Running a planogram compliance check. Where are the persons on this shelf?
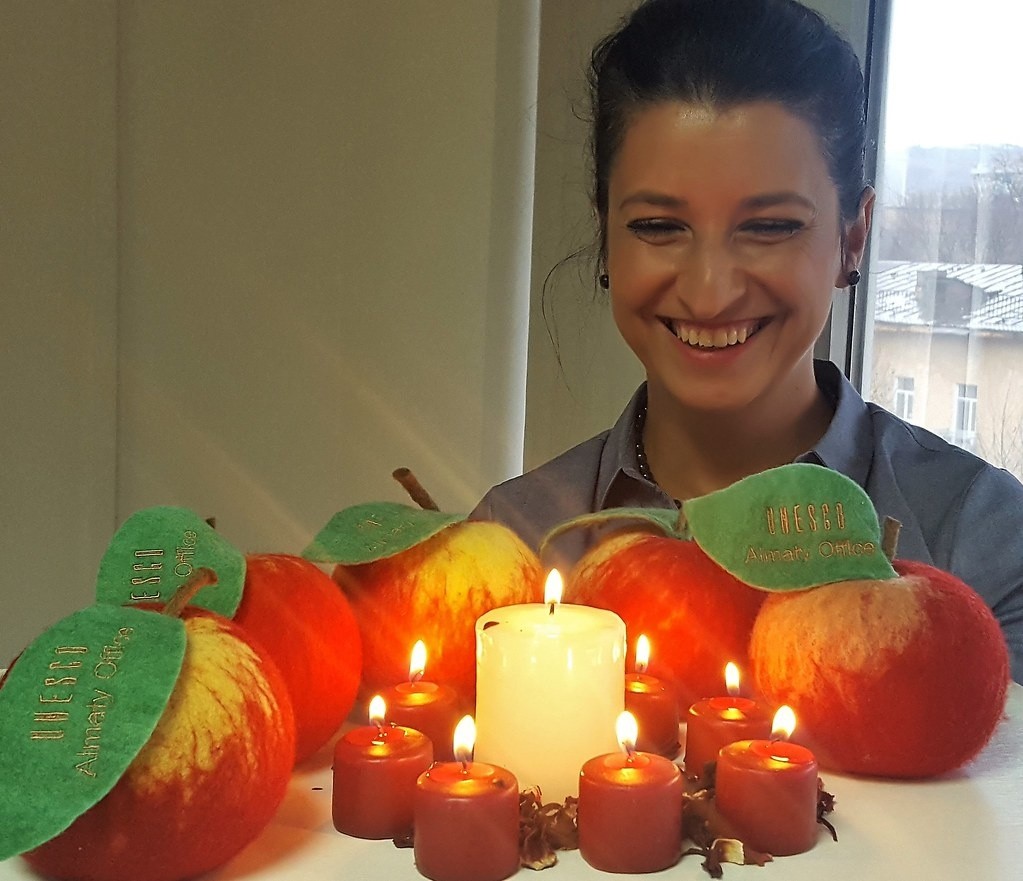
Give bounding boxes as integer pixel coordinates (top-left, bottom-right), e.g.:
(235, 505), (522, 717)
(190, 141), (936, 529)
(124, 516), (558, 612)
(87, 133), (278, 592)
(469, 0), (1023, 685)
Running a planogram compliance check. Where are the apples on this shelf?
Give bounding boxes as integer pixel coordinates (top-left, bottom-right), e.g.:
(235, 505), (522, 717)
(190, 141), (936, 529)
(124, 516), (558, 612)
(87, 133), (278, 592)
(0, 467), (1009, 881)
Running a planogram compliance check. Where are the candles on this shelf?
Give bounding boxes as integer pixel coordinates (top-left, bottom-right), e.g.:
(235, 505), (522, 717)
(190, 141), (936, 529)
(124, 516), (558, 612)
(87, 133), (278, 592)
(687, 661), (770, 782)
(621, 631), (682, 762)
(715, 703), (818, 857)
(578, 710), (685, 874)
(331, 694), (435, 840)
(473, 568), (628, 806)
(381, 638), (450, 760)
(414, 712), (521, 881)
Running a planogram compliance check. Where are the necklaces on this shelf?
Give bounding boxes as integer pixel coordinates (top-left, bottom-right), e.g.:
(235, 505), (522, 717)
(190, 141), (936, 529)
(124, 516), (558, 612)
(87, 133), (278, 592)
(632, 387), (682, 511)
